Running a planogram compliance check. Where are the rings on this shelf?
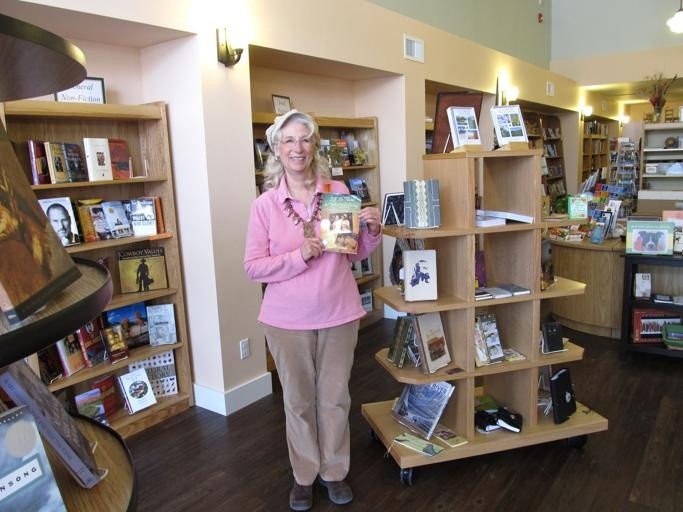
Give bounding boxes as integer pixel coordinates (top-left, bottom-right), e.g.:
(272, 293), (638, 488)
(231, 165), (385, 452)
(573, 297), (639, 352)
(375, 215), (378, 220)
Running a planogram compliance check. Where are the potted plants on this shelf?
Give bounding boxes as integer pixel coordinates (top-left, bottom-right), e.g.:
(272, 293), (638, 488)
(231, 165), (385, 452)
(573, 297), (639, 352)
(643, 73), (678, 122)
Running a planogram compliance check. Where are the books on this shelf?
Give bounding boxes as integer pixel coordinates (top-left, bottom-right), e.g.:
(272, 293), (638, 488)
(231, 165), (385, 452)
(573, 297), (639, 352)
(251, 133), (374, 314)
(116, 248), (168, 293)
(475, 209), (534, 434)
(38, 197), (165, 246)
(550, 148), (639, 244)
(626, 210), (683, 347)
(541, 323), (564, 354)
(27, 302), (177, 385)
(28, 138), (134, 187)
(447, 107), (481, 149)
(539, 238), (554, 291)
(52, 350), (178, 426)
(384, 179), (468, 457)
(524, 119), (568, 214)
(550, 368), (577, 425)
(385, 103), (683, 445)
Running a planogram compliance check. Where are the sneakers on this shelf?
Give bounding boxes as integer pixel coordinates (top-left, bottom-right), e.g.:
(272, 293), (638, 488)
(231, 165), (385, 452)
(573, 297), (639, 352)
(319, 477), (353, 504)
(290, 478), (314, 510)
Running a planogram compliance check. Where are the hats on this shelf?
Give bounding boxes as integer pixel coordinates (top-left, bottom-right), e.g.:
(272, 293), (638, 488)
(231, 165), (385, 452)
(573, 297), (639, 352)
(266, 109), (312, 151)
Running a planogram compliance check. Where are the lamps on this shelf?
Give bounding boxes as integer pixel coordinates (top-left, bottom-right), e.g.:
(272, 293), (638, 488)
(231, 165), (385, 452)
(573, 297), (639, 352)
(666, 0), (683, 34)
(216, 28), (243, 67)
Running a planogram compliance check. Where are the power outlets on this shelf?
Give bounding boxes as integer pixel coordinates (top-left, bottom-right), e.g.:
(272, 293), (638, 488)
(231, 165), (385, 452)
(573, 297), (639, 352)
(240, 338), (250, 360)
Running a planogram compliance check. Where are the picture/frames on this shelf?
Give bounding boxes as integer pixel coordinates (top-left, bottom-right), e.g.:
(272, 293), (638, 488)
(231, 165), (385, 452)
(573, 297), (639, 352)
(381, 193), (403, 227)
(54, 77), (106, 103)
(271, 94), (291, 115)
(443, 107), (482, 153)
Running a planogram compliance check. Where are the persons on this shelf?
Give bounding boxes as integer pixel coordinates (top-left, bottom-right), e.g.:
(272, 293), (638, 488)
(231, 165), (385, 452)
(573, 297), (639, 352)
(243, 108), (384, 511)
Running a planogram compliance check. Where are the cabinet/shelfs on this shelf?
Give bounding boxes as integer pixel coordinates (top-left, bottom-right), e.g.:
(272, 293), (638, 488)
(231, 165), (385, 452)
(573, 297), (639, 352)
(0, 12), (139, 512)
(252, 111), (385, 329)
(522, 112), (567, 196)
(619, 123), (683, 361)
(362, 149), (609, 470)
(582, 115), (619, 183)
(0, 99), (196, 440)
